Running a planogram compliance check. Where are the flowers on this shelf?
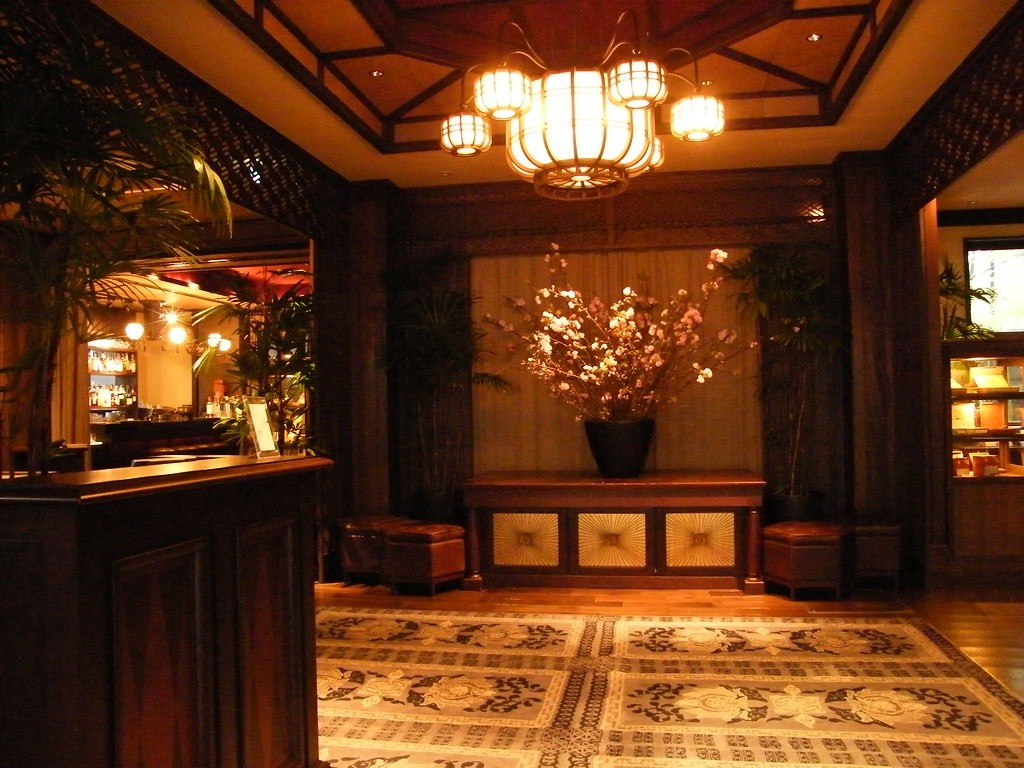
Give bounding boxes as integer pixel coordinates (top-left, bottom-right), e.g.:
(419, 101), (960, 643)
(484, 243), (807, 423)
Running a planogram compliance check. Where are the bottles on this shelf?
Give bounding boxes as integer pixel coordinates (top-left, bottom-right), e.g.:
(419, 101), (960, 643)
(88, 350), (136, 373)
(207, 395), (243, 418)
(89, 384), (136, 406)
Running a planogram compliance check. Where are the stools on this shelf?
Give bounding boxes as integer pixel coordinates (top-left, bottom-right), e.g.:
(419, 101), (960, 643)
(855, 512), (908, 607)
(337, 513), (410, 587)
(761, 521), (855, 600)
(383, 524), (467, 596)
(149, 442), (229, 455)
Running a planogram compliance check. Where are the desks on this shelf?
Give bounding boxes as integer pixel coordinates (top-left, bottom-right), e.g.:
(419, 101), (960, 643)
(0, 453), (335, 768)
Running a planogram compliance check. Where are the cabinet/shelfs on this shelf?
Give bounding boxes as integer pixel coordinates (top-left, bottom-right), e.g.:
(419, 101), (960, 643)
(944, 338), (1024, 587)
(86, 312), (147, 421)
(463, 470), (766, 595)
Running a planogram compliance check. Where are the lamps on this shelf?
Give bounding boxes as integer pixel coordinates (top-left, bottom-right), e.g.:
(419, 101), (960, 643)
(123, 300), (132, 312)
(106, 298), (113, 309)
(439, 10), (725, 202)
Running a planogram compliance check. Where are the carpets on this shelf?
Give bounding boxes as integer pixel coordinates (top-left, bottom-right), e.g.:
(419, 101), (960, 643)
(315, 607), (1024, 768)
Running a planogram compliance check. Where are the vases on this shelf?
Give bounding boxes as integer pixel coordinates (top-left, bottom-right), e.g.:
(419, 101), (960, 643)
(584, 417), (656, 478)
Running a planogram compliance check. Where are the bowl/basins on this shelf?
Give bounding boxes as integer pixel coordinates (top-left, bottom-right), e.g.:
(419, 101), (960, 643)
(134, 405), (192, 419)
(105, 412), (117, 420)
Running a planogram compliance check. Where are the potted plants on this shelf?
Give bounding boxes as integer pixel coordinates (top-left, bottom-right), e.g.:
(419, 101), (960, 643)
(713, 243), (848, 522)
(938, 250), (998, 340)
(381, 289), (522, 523)
